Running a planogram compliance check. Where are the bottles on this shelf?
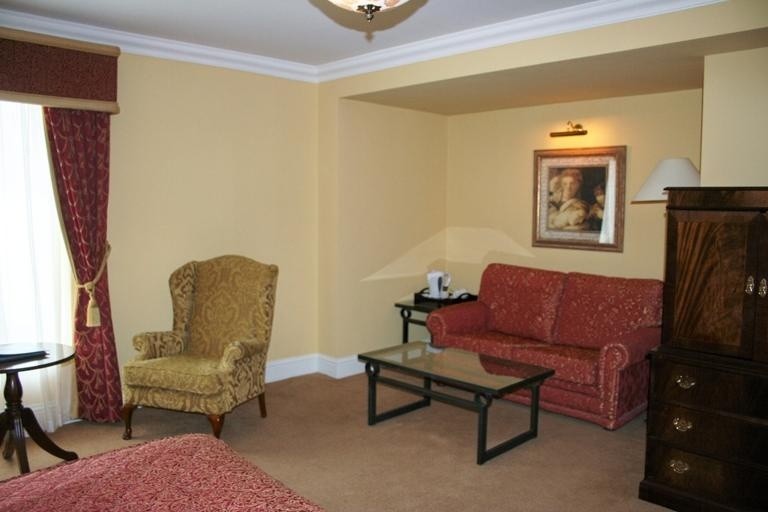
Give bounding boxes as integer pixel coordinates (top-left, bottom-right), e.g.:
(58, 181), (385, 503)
(452, 288), (468, 299)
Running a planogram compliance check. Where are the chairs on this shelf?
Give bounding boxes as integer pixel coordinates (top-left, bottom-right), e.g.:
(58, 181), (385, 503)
(120, 255), (279, 439)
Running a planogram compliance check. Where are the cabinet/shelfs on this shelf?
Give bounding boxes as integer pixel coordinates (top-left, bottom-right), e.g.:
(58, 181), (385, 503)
(661, 186), (767, 364)
(639, 348), (768, 512)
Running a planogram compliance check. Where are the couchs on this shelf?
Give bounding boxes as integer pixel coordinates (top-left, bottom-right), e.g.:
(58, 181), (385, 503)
(424, 264), (665, 431)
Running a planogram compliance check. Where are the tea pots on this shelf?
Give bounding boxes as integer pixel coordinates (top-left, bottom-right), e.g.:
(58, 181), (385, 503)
(426, 270), (452, 298)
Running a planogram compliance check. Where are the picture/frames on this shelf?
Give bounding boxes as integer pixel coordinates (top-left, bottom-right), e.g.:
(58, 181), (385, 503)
(532, 146), (626, 254)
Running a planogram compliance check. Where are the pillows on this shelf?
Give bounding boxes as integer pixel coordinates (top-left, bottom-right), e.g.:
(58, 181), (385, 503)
(552, 273), (664, 352)
(476, 265), (566, 346)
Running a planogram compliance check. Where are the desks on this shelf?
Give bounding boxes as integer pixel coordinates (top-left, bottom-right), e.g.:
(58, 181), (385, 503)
(0, 341), (77, 474)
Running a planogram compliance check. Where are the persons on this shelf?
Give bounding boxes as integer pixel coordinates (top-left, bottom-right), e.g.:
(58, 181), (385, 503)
(546, 168), (605, 232)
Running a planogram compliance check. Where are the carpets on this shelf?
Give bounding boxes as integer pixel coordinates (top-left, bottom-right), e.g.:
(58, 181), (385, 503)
(0, 431), (333, 512)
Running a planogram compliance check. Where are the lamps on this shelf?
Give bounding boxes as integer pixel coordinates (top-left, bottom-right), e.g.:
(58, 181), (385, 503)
(550, 121), (587, 136)
(327, 0), (409, 24)
(630, 157), (700, 203)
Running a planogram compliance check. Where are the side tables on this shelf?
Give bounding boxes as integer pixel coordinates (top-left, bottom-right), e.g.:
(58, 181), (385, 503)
(393, 294), (478, 343)
